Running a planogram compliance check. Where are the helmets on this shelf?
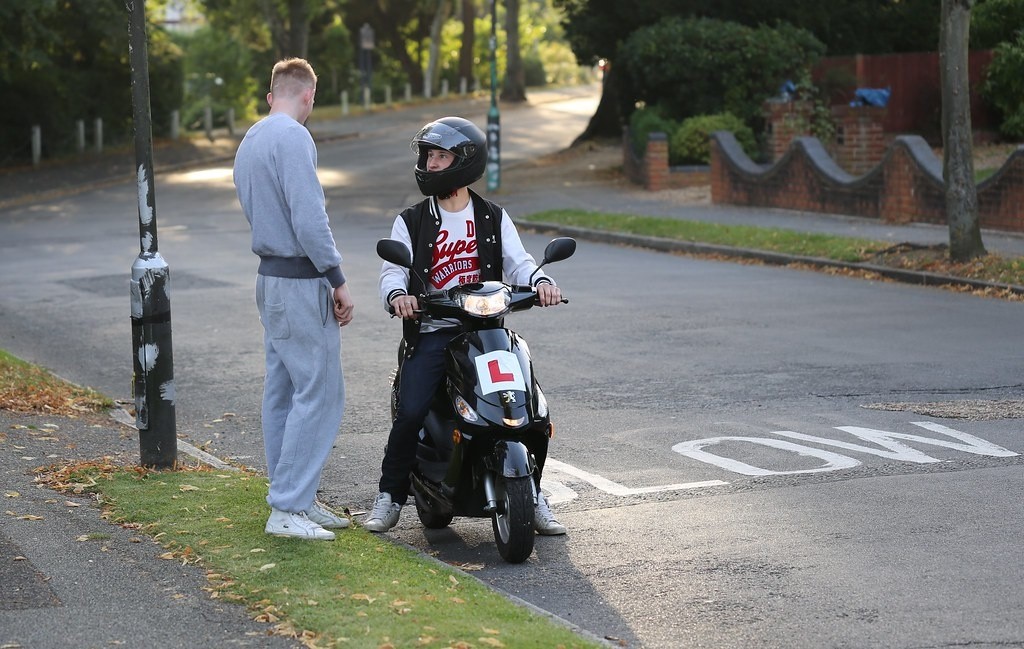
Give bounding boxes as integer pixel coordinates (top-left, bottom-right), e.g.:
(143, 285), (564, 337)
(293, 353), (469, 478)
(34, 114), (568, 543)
(410, 117), (488, 200)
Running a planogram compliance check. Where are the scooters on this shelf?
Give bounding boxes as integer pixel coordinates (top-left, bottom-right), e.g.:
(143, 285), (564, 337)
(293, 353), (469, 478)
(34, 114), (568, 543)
(375, 235), (579, 562)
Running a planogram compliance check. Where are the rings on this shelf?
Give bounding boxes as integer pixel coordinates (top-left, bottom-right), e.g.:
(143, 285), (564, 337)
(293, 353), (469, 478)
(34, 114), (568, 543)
(405, 301), (410, 305)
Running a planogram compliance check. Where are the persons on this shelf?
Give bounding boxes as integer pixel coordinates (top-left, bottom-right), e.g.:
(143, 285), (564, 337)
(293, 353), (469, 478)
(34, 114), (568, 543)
(233, 56), (352, 540)
(366, 116), (566, 536)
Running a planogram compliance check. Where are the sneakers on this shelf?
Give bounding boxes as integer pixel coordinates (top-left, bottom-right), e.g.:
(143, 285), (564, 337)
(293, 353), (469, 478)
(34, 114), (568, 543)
(307, 494), (350, 529)
(535, 492), (566, 535)
(363, 492), (402, 532)
(264, 506), (335, 540)
